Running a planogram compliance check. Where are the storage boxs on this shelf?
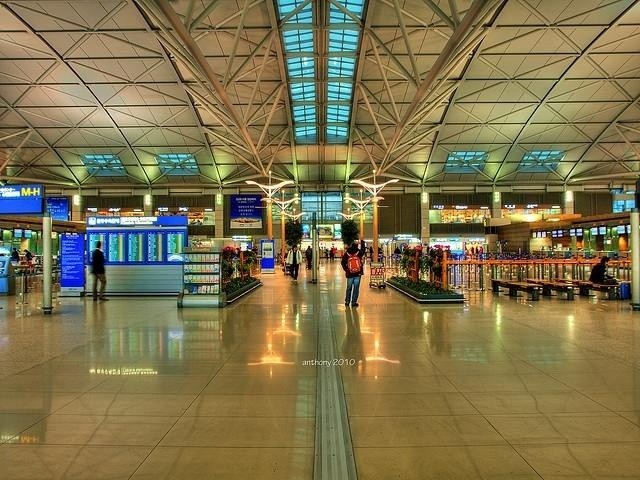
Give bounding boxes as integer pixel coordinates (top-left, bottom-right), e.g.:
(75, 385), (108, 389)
(619, 282), (632, 299)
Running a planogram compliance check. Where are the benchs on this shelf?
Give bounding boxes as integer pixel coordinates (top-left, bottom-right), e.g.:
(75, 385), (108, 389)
(490, 278), (620, 301)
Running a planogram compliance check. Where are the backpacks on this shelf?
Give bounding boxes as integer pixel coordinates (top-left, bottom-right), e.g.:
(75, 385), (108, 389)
(347, 251), (362, 273)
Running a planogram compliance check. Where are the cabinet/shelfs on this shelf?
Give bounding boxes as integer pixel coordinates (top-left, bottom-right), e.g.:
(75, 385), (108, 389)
(180, 251), (222, 296)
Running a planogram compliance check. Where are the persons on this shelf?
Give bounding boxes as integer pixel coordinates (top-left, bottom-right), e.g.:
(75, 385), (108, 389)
(12, 247), (19, 262)
(279, 244), (484, 271)
(89, 240), (110, 301)
(286, 244), (302, 283)
(588, 257), (617, 285)
(23, 247), (34, 262)
(342, 243), (365, 307)
